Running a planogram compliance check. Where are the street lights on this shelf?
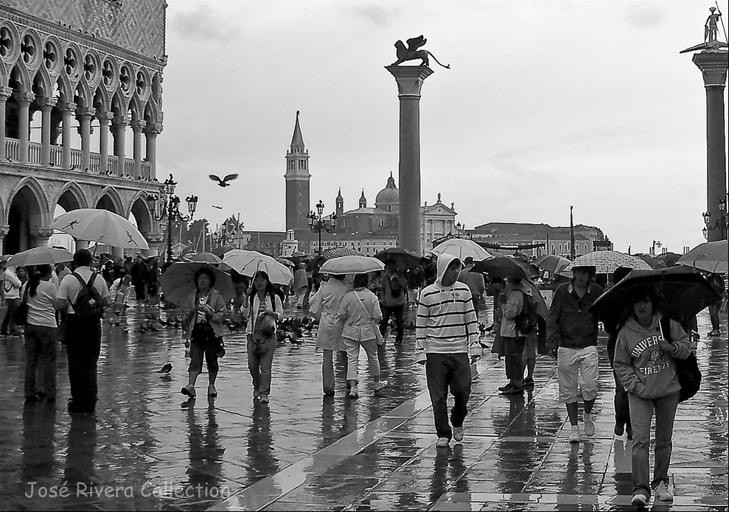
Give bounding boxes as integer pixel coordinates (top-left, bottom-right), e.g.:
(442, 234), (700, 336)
(306, 199), (339, 254)
(147, 173), (199, 300)
(447, 222), (471, 240)
(699, 198), (729, 242)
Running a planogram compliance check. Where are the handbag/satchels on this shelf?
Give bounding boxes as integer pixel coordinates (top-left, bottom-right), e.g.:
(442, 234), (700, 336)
(13, 301), (29, 327)
(373, 321), (386, 346)
(665, 350), (702, 403)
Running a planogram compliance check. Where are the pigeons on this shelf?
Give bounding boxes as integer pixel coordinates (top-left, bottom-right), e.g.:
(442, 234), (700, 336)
(478, 340), (491, 349)
(212, 206), (222, 209)
(157, 363), (172, 375)
(209, 173), (240, 187)
(139, 312), (320, 344)
(484, 323), (494, 333)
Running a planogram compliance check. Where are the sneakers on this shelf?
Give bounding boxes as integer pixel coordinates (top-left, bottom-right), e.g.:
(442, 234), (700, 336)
(569, 413), (674, 505)
(348, 388), (358, 399)
(436, 435), (450, 447)
(259, 393), (270, 404)
(181, 385), (196, 397)
(207, 384), (218, 397)
(252, 388), (261, 398)
(374, 379), (388, 391)
(497, 377), (534, 396)
(706, 327), (722, 336)
(394, 340), (402, 346)
(453, 424), (463, 442)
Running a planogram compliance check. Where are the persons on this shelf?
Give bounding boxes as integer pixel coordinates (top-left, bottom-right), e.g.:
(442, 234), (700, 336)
(704, 7), (722, 42)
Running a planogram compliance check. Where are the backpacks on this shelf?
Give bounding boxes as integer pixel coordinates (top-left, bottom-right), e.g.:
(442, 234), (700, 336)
(66, 270), (104, 323)
(508, 288), (538, 335)
